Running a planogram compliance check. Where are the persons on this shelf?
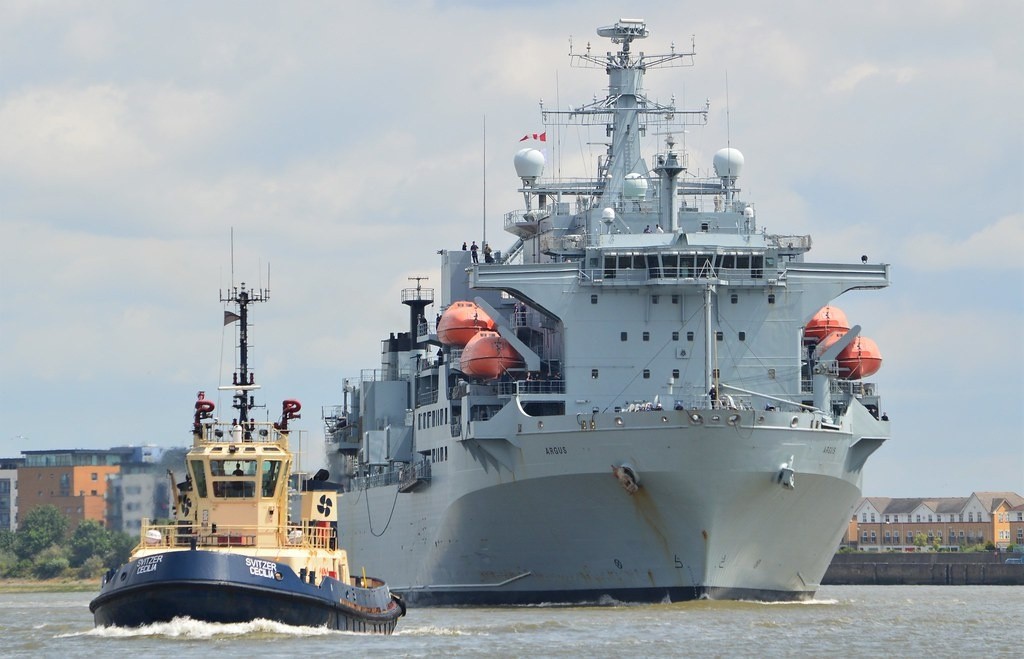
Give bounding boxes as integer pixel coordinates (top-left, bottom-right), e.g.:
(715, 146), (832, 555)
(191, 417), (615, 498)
(881, 412), (888, 421)
(437, 349), (443, 366)
(484, 244), (492, 263)
(435, 314), (441, 328)
(709, 384), (717, 409)
(470, 241), (479, 263)
(676, 404), (683, 410)
(870, 409), (879, 420)
(629, 400), (662, 411)
(461, 241), (467, 250)
(418, 314), (427, 335)
(502, 370), (562, 394)
(643, 224), (664, 233)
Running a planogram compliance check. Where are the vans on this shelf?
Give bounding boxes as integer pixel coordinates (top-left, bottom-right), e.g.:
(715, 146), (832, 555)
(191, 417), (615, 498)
(1004, 558), (1021, 564)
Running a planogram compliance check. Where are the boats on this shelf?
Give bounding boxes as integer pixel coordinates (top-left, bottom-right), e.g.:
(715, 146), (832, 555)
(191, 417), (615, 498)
(90, 273), (404, 634)
(328, 20), (892, 606)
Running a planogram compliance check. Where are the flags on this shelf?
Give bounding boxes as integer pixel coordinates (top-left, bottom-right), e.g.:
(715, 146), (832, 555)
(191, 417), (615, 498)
(520, 132), (546, 141)
